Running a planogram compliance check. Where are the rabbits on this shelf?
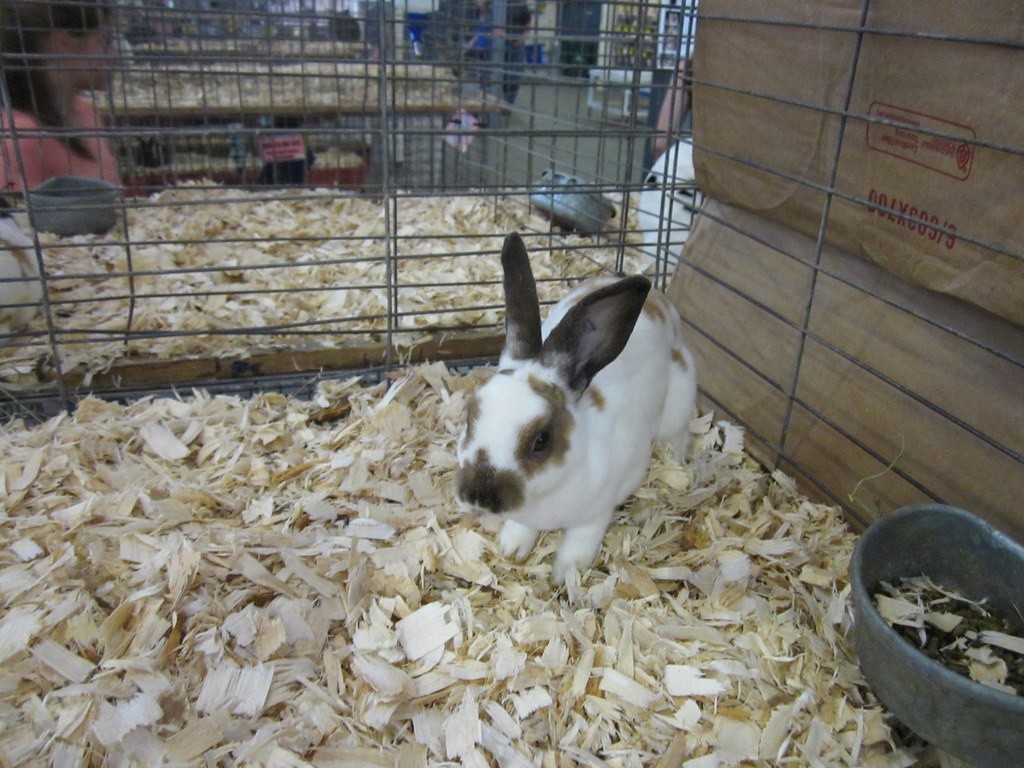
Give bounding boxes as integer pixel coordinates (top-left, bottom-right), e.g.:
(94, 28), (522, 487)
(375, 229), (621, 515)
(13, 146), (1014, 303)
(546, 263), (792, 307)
(642, 139), (706, 253)
(457, 230), (699, 587)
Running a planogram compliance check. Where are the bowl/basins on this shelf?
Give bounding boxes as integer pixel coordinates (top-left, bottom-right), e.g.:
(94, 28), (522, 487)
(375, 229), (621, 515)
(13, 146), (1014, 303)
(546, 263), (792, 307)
(531, 168), (617, 233)
(848, 503), (1024, 768)
(28, 175), (117, 235)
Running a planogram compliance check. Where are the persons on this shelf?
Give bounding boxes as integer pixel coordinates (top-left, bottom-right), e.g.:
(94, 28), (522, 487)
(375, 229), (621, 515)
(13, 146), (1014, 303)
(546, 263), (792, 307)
(0, 0), (120, 200)
(475, 0), (532, 107)
(652, 48), (693, 148)
